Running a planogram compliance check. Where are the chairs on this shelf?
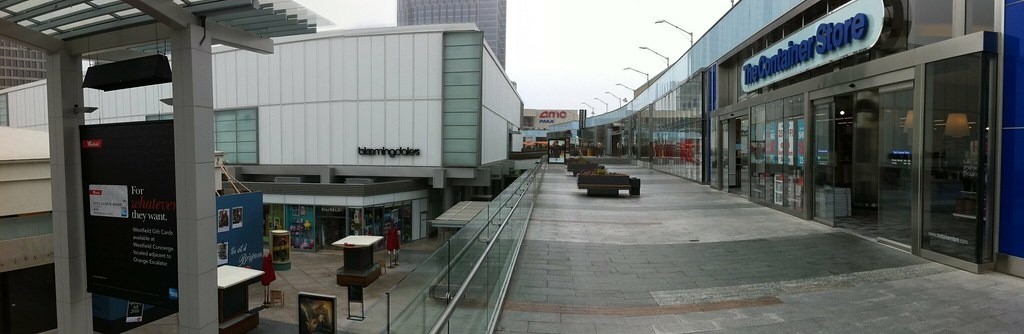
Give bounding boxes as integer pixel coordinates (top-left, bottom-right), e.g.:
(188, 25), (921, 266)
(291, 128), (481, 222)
(270, 290), (284, 308)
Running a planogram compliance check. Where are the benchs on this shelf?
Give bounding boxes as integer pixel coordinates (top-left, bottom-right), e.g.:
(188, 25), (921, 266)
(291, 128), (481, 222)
(567, 162), (631, 196)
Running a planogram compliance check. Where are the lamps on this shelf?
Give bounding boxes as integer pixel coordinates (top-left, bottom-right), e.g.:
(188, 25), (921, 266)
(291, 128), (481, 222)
(944, 113), (970, 165)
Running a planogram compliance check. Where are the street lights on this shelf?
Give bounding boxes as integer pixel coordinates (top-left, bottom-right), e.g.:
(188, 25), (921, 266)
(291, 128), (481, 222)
(605, 92), (621, 107)
(581, 102), (594, 116)
(655, 19), (693, 47)
(639, 46), (670, 68)
(616, 83), (635, 92)
(593, 97), (608, 113)
(622, 67), (649, 81)
